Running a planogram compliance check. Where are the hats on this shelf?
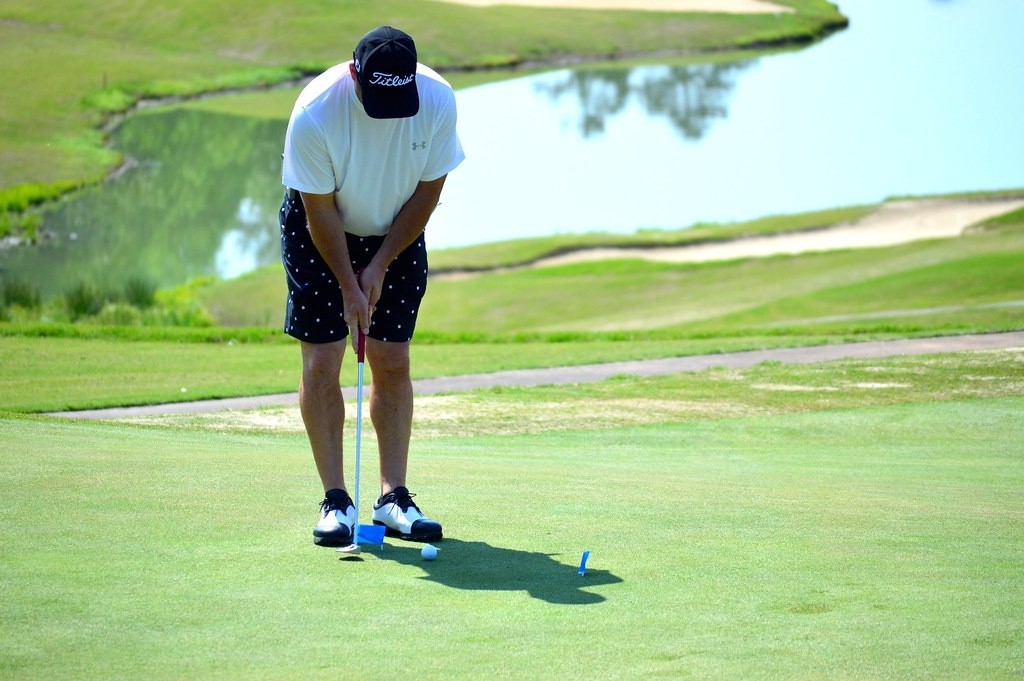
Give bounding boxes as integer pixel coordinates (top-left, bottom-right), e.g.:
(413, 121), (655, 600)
(353, 25), (420, 119)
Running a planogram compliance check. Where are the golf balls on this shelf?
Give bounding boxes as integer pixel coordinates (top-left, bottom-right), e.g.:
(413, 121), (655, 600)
(420, 543), (438, 562)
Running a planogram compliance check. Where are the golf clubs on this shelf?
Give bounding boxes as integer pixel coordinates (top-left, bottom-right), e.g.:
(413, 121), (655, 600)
(334, 323), (367, 556)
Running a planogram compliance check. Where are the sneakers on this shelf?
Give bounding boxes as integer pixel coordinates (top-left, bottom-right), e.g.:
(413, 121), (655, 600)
(312, 489), (355, 547)
(372, 486), (443, 542)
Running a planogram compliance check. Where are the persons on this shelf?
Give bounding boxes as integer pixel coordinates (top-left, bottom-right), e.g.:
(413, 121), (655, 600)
(276, 25), (468, 547)
